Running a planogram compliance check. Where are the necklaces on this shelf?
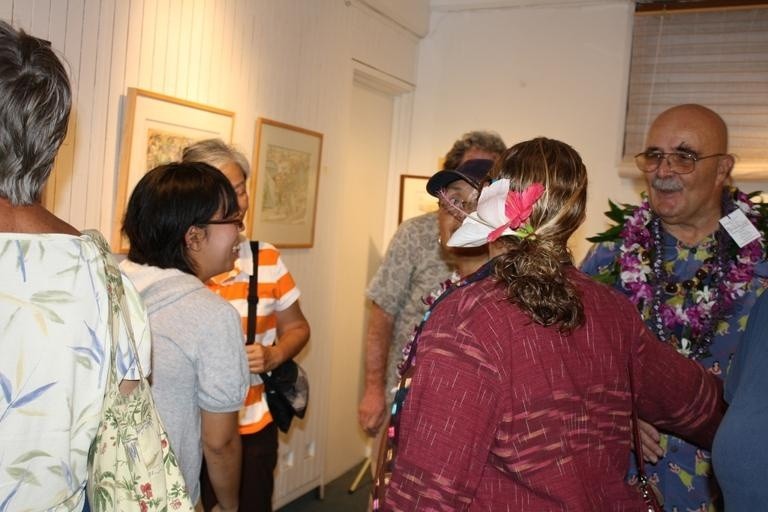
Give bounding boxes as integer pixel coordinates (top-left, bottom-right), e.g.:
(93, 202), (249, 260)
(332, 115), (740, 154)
(396, 276), (466, 369)
(619, 185), (767, 358)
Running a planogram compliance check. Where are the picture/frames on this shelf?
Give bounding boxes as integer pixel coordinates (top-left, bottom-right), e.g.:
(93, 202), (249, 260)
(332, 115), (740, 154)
(112, 86), (324, 255)
(398, 173), (440, 225)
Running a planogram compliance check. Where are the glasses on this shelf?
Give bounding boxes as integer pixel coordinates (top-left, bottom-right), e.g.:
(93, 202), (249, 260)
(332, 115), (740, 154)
(633, 150), (724, 177)
(205, 217), (245, 229)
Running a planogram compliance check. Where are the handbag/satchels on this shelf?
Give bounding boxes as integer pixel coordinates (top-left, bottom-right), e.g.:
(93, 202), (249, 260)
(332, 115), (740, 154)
(245, 240), (309, 434)
(83, 230), (195, 511)
(628, 355), (661, 512)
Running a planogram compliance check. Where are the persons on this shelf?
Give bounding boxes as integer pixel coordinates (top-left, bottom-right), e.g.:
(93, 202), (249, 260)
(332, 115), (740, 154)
(182, 135), (312, 511)
(371, 158), (499, 512)
(359, 130), (507, 476)
(0, 20), (153, 512)
(578, 103), (768, 512)
(713, 291), (768, 512)
(115, 161), (253, 511)
(384, 137), (728, 512)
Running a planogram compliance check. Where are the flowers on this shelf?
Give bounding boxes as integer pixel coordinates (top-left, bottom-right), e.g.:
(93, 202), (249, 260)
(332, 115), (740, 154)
(436, 177), (545, 250)
(585, 178), (767, 363)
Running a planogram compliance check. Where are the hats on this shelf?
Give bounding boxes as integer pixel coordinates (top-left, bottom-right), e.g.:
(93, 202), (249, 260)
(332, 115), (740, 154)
(425, 158), (492, 198)
